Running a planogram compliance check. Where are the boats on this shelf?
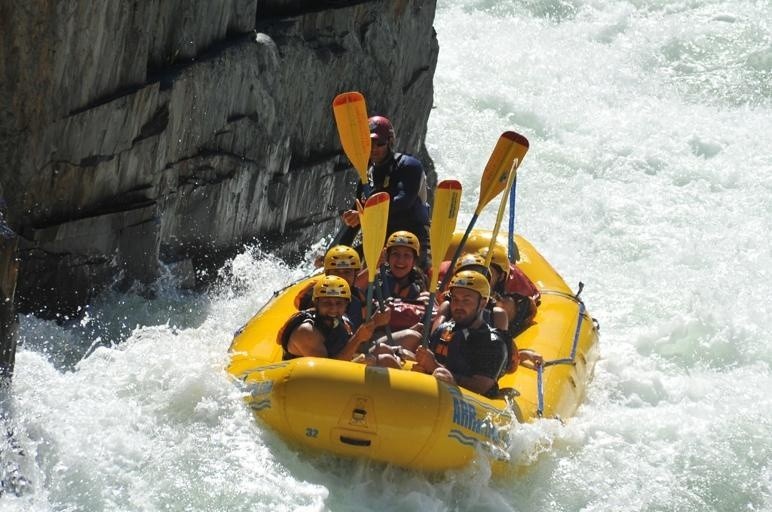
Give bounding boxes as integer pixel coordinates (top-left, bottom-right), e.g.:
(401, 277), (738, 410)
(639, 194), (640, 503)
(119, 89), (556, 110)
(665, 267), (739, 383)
(225, 231), (601, 485)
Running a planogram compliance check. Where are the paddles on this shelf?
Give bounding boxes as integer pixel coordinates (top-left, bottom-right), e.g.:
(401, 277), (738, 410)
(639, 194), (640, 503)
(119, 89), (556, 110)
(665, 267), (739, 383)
(438, 130), (529, 293)
(355, 197), (394, 346)
(422, 180), (462, 349)
(363, 191), (390, 355)
(333, 92), (391, 297)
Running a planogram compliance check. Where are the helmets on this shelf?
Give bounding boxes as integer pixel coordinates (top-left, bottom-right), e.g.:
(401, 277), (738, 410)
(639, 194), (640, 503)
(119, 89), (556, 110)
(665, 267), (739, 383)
(312, 274), (353, 306)
(386, 230), (421, 257)
(364, 114), (396, 146)
(450, 245), (510, 317)
(323, 243), (362, 274)
